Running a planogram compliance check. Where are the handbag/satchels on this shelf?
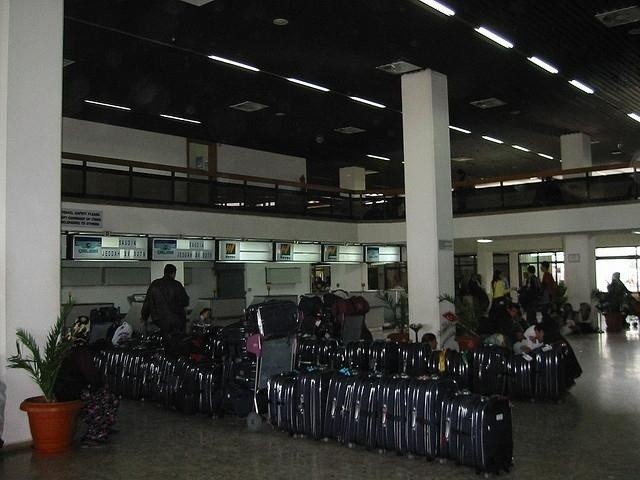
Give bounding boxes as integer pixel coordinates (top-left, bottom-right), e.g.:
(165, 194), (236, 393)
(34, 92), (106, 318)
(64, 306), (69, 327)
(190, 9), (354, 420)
(245, 289), (371, 341)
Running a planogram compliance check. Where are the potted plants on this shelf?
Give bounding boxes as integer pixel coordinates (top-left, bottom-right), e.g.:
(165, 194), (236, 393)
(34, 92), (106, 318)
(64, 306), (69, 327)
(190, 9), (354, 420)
(373, 287), (411, 342)
(434, 280), (487, 355)
(591, 281), (629, 332)
(1, 290), (98, 456)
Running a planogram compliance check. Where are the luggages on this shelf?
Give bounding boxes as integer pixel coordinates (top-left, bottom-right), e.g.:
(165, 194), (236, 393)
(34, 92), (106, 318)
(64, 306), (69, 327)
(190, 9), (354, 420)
(93, 323), (261, 418)
(267, 373), (515, 478)
(291, 336), (560, 404)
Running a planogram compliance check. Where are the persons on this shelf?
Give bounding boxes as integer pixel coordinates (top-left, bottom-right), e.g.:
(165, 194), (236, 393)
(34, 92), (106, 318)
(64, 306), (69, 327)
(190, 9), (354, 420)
(607, 272), (632, 294)
(141, 264), (190, 339)
(192, 308), (215, 336)
(421, 261), (594, 395)
(454, 169), (475, 191)
